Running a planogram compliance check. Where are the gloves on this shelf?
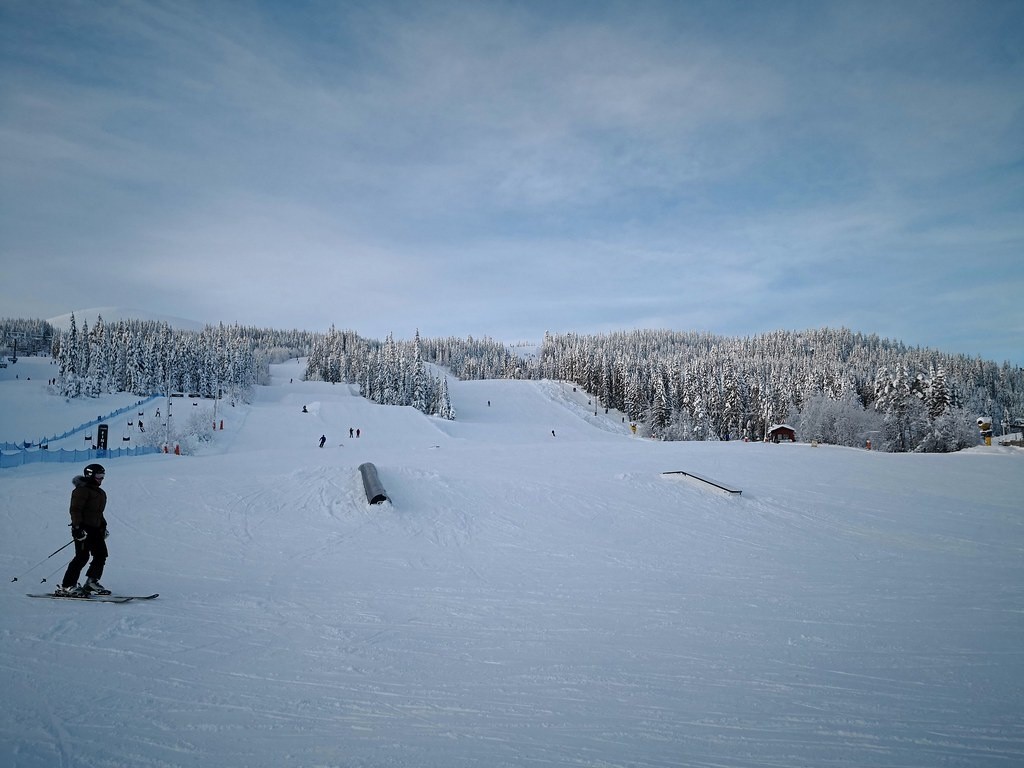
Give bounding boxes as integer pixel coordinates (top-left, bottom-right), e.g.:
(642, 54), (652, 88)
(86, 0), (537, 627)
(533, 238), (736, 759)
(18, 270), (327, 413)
(104, 529), (110, 539)
(73, 526), (89, 542)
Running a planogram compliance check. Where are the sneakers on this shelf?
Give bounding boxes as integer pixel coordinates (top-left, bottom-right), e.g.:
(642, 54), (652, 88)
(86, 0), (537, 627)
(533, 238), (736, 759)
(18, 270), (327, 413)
(61, 586), (86, 596)
(83, 577), (111, 595)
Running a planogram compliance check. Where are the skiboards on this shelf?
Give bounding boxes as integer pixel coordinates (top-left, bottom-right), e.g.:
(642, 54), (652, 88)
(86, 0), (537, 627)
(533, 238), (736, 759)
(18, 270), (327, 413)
(24, 592), (161, 604)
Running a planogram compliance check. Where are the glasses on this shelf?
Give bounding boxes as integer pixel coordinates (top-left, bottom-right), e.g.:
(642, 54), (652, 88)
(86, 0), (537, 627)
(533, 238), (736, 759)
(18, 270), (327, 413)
(95, 473), (105, 479)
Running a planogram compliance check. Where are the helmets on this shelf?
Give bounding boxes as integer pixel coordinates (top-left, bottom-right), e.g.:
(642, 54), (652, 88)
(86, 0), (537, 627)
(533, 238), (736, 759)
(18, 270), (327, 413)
(84, 464), (106, 479)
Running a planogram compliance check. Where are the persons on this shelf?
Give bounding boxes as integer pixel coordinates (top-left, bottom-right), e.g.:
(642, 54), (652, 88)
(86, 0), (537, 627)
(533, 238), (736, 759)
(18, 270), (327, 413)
(319, 435), (326, 447)
(138, 420), (145, 432)
(155, 408), (161, 417)
(231, 399), (234, 407)
(54, 464), (112, 598)
(349, 428), (354, 438)
(356, 429), (360, 438)
